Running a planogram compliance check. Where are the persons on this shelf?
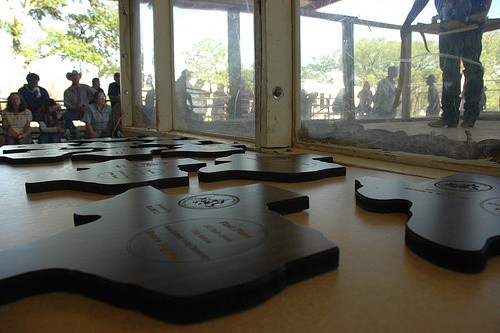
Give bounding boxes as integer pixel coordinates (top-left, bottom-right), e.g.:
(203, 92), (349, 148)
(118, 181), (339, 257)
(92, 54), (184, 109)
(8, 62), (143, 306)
(58, 70), (98, 141)
(18, 71), (50, 121)
(83, 91), (112, 139)
(178, 69), (194, 88)
(424, 74), (441, 116)
(175, 78), (194, 114)
(427, 0), (492, 129)
(374, 66), (399, 119)
(144, 74), (155, 110)
(88, 78), (107, 104)
(36, 98), (65, 144)
(192, 79), (210, 120)
(211, 83), (230, 122)
(2, 92), (33, 145)
(356, 81), (374, 118)
(107, 72), (121, 107)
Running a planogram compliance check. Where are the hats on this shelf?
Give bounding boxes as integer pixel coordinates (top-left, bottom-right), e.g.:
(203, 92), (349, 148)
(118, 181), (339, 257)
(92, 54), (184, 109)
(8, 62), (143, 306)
(424, 74), (437, 83)
(178, 69), (194, 80)
(114, 73), (120, 80)
(66, 70), (82, 81)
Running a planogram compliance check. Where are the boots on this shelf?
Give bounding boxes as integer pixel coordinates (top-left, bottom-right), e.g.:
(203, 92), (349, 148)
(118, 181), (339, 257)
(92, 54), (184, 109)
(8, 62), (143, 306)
(462, 68), (484, 127)
(426, 70), (461, 127)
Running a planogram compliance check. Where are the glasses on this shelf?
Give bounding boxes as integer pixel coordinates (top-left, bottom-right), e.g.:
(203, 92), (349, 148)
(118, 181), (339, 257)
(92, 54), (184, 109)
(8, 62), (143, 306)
(96, 96), (106, 99)
